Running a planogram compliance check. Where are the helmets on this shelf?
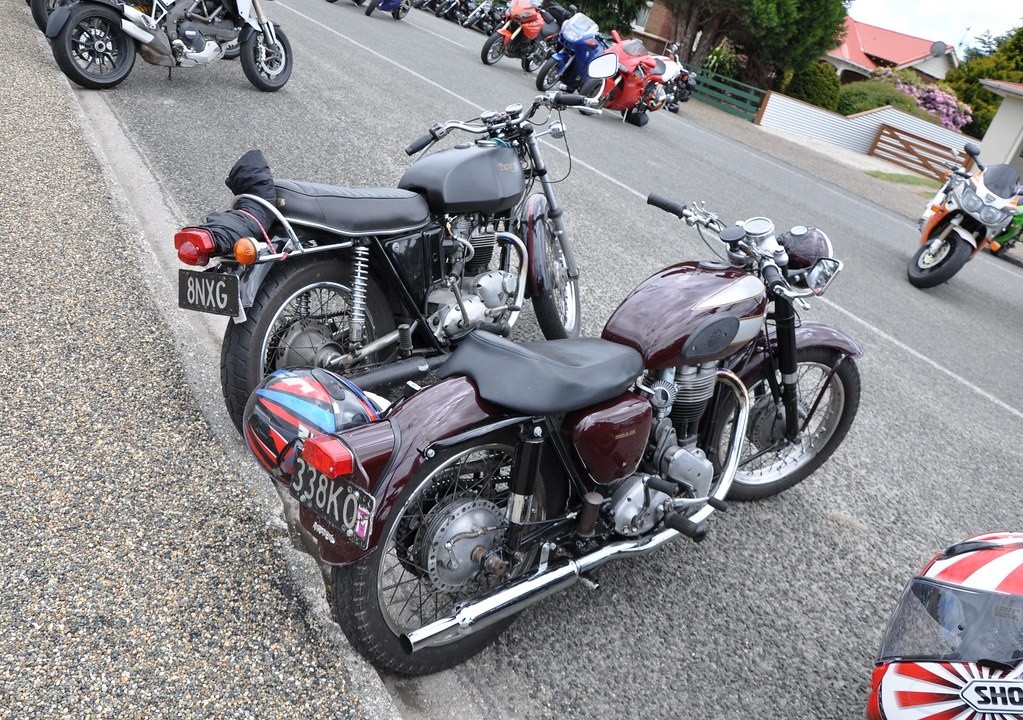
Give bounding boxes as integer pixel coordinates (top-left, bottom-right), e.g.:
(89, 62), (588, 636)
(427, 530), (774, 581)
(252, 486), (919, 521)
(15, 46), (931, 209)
(866, 531), (1023, 720)
(646, 84), (666, 112)
(242, 366), (383, 488)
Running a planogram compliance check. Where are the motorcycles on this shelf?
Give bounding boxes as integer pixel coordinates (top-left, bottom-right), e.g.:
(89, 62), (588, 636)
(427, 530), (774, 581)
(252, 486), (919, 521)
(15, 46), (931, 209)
(26, 0), (293, 93)
(244, 197), (861, 680)
(906, 142), (1023, 292)
(359, 2), (700, 128)
(168, 88), (593, 443)
